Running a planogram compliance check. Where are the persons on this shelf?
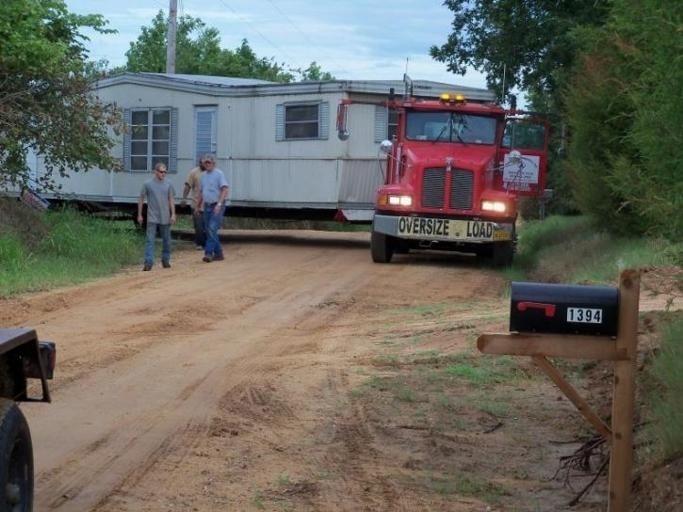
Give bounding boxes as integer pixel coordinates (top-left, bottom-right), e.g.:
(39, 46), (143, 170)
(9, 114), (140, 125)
(180, 148), (214, 250)
(137, 163), (176, 271)
(193, 156), (229, 262)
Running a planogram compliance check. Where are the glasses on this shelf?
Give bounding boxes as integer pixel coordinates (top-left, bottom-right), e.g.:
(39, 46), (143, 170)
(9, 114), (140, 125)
(157, 169), (168, 174)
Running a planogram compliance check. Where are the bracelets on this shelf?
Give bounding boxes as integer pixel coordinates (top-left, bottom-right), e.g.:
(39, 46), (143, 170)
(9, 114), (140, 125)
(217, 202), (224, 208)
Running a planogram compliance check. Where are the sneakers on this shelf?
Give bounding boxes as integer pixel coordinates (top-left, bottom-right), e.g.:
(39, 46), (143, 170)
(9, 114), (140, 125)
(195, 245), (203, 250)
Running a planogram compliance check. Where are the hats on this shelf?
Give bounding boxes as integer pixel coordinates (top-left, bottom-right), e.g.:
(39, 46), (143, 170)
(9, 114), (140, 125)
(202, 154), (216, 164)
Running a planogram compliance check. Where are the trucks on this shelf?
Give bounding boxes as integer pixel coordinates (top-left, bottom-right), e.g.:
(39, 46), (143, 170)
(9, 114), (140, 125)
(336, 96), (550, 263)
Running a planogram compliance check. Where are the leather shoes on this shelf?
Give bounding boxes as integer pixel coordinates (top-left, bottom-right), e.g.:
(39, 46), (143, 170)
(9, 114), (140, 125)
(142, 265), (152, 271)
(163, 263), (171, 268)
(202, 254), (224, 262)
